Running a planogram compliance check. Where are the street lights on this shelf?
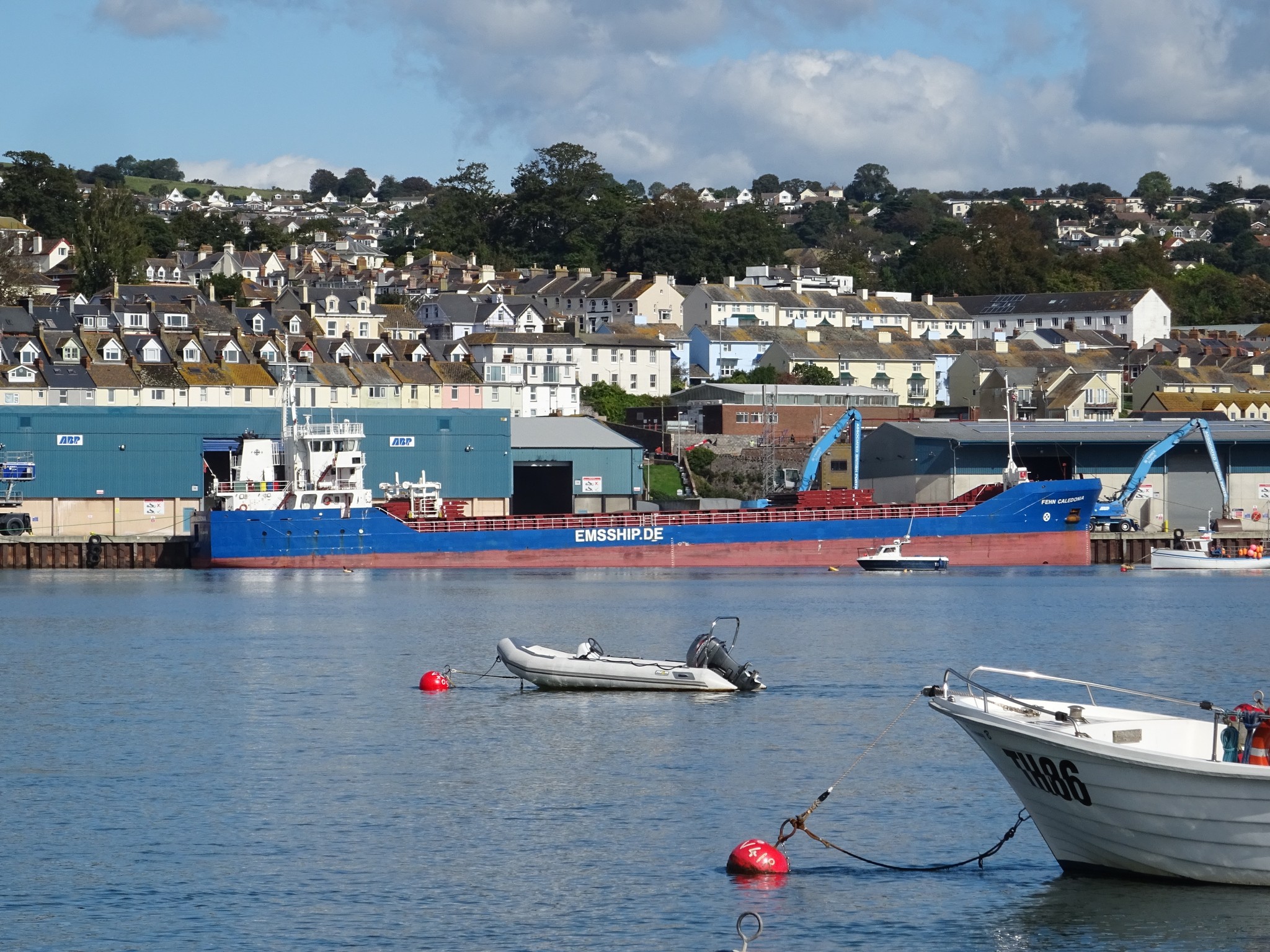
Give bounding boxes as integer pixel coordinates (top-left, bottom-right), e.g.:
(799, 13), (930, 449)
(678, 411), (683, 464)
(644, 458), (650, 501)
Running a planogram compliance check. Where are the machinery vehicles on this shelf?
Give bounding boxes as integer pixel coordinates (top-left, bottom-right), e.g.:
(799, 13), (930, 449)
(1088, 418), (1229, 532)
(740, 406), (862, 509)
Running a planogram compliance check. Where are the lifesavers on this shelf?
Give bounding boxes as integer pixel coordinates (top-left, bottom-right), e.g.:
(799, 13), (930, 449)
(1253, 513), (1260, 520)
(1229, 702), (1265, 763)
(1249, 706), (1270, 766)
(1174, 543), (1184, 550)
(89, 535), (101, 546)
(90, 554), (101, 563)
(240, 504), (247, 511)
(90, 546), (102, 554)
(1174, 529), (1184, 538)
(324, 497), (331, 505)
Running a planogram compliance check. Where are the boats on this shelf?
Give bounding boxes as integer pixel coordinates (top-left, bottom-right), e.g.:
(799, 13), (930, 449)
(855, 513), (949, 571)
(211, 335), (1102, 568)
(921, 666), (1270, 891)
(497, 616), (767, 693)
(1150, 506), (1270, 569)
